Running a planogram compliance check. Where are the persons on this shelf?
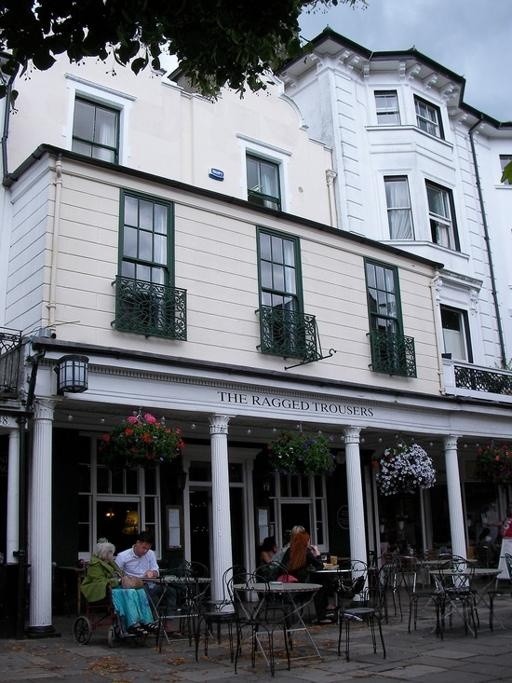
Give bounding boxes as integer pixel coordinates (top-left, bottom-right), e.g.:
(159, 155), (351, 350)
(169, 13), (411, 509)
(266, 524), (308, 582)
(257, 535), (277, 567)
(376, 521), (391, 565)
(393, 519), (412, 568)
(114, 530), (183, 638)
(493, 502), (511, 527)
(274, 530), (344, 625)
(478, 526), (500, 569)
(79, 540), (160, 636)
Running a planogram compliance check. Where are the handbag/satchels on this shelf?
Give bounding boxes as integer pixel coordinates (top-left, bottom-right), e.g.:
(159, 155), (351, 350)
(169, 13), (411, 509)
(120, 575), (144, 590)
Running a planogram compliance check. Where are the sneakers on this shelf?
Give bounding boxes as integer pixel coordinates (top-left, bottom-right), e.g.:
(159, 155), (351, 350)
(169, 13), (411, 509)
(144, 622), (160, 632)
(128, 625), (148, 637)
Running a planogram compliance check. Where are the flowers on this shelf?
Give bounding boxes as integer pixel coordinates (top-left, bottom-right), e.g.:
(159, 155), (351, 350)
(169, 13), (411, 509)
(269, 428), (336, 476)
(104, 410), (186, 466)
(468, 441), (512, 484)
(375, 440), (438, 497)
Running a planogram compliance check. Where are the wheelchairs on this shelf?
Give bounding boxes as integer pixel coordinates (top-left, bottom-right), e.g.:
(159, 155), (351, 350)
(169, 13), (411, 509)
(73, 558), (151, 648)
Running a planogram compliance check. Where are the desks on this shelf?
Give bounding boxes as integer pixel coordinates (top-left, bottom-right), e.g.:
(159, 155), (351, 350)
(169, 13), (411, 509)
(136, 575), (215, 644)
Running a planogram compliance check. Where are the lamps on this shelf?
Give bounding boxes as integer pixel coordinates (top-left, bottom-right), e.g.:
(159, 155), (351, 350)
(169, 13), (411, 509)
(54, 353), (88, 396)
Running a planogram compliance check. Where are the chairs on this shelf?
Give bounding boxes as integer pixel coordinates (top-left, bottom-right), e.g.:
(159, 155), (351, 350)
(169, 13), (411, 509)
(156, 545), (512, 677)
